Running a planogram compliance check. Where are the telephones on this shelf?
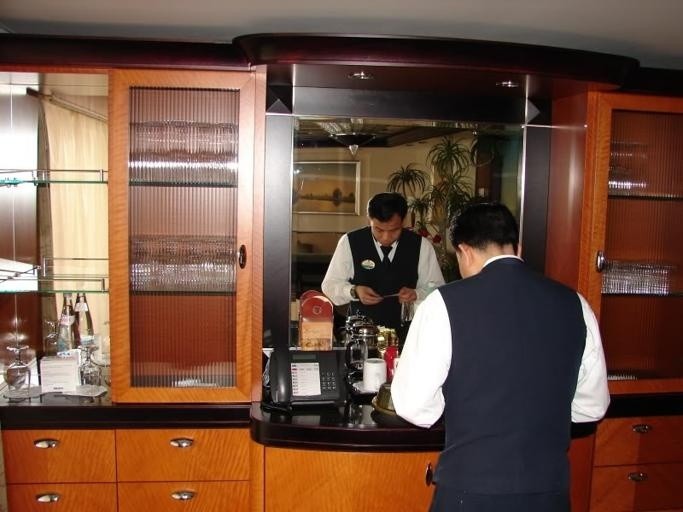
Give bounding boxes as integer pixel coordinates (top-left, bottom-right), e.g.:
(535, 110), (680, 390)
(260, 347), (347, 413)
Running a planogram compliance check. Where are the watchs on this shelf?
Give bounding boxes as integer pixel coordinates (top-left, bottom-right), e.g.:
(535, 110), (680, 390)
(350, 285), (359, 299)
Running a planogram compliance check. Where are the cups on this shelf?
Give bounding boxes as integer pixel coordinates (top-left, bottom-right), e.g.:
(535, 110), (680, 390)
(77, 345), (101, 391)
(376, 382), (402, 411)
(364, 358), (386, 390)
(5, 345), (30, 396)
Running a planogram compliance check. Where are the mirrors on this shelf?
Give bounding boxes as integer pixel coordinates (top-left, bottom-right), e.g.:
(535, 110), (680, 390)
(288, 115), (525, 359)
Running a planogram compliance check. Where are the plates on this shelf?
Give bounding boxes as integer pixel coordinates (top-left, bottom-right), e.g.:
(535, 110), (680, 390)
(371, 396), (397, 417)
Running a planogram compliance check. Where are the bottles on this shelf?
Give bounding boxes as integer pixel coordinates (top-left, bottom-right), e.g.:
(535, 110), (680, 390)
(57, 293), (80, 355)
(74, 293), (94, 348)
(384, 336), (398, 384)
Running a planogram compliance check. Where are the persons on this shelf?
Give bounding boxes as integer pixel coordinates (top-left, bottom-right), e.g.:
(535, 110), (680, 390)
(319, 192), (445, 346)
(390, 202), (611, 512)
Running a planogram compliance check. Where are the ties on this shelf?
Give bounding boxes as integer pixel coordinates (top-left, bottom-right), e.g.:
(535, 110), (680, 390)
(381, 245), (392, 265)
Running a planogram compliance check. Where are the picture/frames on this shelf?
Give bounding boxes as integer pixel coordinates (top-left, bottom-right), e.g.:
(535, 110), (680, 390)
(293, 159), (361, 217)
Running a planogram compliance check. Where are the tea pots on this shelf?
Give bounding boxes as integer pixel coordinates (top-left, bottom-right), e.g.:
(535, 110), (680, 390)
(346, 316), (381, 373)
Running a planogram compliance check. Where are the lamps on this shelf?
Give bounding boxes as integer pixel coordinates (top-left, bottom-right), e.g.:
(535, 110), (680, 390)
(313, 118), (384, 160)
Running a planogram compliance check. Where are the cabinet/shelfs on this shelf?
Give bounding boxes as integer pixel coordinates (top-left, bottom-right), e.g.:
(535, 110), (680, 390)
(0, 424), (118, 512)
(263, 445), (449, 511)
(568, 413), (682, 511)
(111, 64), (265, 407)
(0, 63), (118, 392)
(544, 91), (681, 398)
(113, 401), (255, 511)
